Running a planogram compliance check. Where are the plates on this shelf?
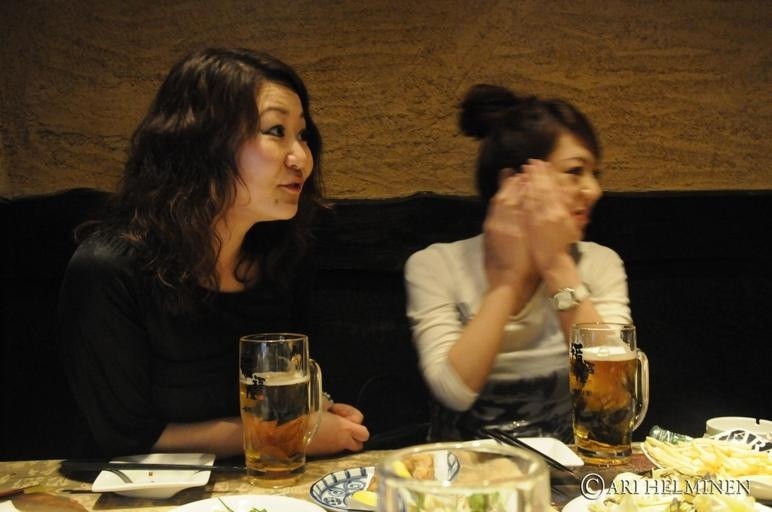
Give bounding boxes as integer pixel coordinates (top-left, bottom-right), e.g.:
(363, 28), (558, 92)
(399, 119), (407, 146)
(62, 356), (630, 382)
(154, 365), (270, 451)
(168, 493), (328, 512)
(310, 465), (406, 512)
(640, 439), (772, 501)
(560, 484), (772, 512)
(91, 452), (216, 501)
(460, 436), (585, 477)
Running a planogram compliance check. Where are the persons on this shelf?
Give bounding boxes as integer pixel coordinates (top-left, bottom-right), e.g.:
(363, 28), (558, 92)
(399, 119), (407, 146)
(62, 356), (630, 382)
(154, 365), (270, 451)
(404, 79), (631, 440)
(51, 45), (420, 458)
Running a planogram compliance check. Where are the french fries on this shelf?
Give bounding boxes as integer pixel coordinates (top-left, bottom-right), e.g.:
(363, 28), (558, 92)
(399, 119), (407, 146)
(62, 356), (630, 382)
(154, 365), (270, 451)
(643, 436), (772, 479)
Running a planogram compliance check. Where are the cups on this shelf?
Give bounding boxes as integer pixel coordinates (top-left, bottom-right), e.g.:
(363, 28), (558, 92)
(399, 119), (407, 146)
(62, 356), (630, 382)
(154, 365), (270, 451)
(238, 331), (323, 480)
(568, 320), (650, 467)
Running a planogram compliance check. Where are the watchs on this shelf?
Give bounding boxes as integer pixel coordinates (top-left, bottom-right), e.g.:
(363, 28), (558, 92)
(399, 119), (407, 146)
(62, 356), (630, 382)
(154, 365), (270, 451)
(553, 286), (587, 310)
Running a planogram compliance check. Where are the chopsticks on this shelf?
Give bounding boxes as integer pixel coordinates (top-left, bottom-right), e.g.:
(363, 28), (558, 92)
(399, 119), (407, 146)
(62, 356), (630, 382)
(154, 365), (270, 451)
(60, 461), (251, 473)
(477, 425), (575, 474)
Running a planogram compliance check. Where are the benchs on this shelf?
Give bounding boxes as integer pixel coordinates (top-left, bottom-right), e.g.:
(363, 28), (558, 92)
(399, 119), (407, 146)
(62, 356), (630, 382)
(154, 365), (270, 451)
(1, 188), (772, 459)
